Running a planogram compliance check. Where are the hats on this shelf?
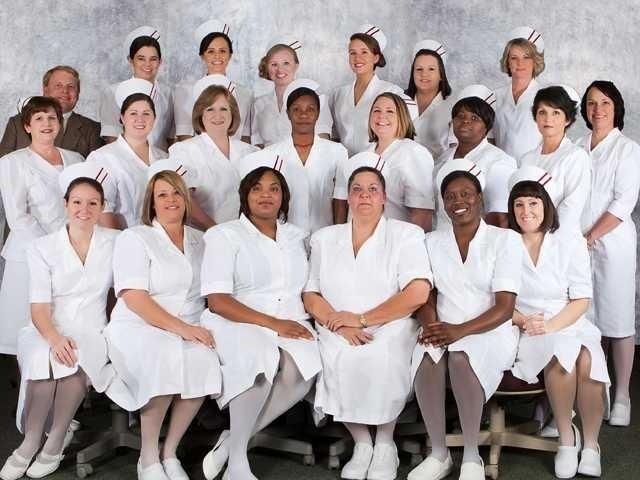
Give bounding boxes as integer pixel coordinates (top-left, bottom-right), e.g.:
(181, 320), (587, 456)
(113, 78), (160, 109)
(342, 149), (393, 195)
(263, 36), (305, 65)
(370, 85), (421, 132)
(541, 82), (582, 112)
(191, 74), (239, 106)
(283, 77), (326, 110)
(146, 160), (191, 186)
(58, 162), (117, 207)
(436, 157), (487, 198)
(452, 83), (498, 118)
(15, 95), (33, 113)
(500, 26), (544, 56)
(412, 38), (448, 67)
(234, 150), (296, 198)
(193, 20), (237, 55)
(353, 23), (388, 53)
(122, 26), (165, 65)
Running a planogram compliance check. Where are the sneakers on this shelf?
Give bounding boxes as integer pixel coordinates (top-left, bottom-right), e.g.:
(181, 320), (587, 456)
(26, 437), (66, 479)
(554, 421), (581, 479)
(458, 455), (485, 480)
(221, 466), (257, 480)
(406, 448), (453, 480)
(0, 438), (43, 480)
(541, 409), (576, 436)
(135, 456), (169, 480)
(608, 396), (632, 426)
(578, 439), (602, 477)
(367, 441), (400, 480)
(160, 451), (189, 480)
(202, 428), (230, 480)
(341, 441), (372, 480)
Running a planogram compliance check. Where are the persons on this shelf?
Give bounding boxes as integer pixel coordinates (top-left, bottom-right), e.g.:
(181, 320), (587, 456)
(519, 86), (593, 437)
(330, 23), (406, 157)
(579, 82), (639, 427)
(302, 165), (433, 480)
(407, 171), (522, 480)
(250, 79), (348, 246)
(406, 39), (454, 161)
(1, 65), (100, 241)
(426, 84), (519, 231)
(118, 25), (174, 153)
(87, 77), (169, 230)
(1, 182), (126, 480)
(173, 18), (252, 145)
(252, 36), (335, 150)
(343, 92), (434, 236)
(0, 97), (86, 430)
(492, 28), (543, 162)
(105, 169), (222, 480)
(171, 74), (261, 233)
(200, 165), (324, 480)
(505, 182), (612, 480)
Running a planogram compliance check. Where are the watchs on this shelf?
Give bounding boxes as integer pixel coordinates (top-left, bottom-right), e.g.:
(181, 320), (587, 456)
(360, 314), (367, 329)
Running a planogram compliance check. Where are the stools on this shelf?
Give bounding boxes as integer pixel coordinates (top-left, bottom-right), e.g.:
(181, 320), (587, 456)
(328, 399), (426, 470)
(428, 374), (568, 478)
(73, 390), (169, 476)
(246, 418), (319, 468)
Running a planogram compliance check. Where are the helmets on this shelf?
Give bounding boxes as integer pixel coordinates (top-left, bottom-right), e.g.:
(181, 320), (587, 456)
(507, 166), (561, 209)
